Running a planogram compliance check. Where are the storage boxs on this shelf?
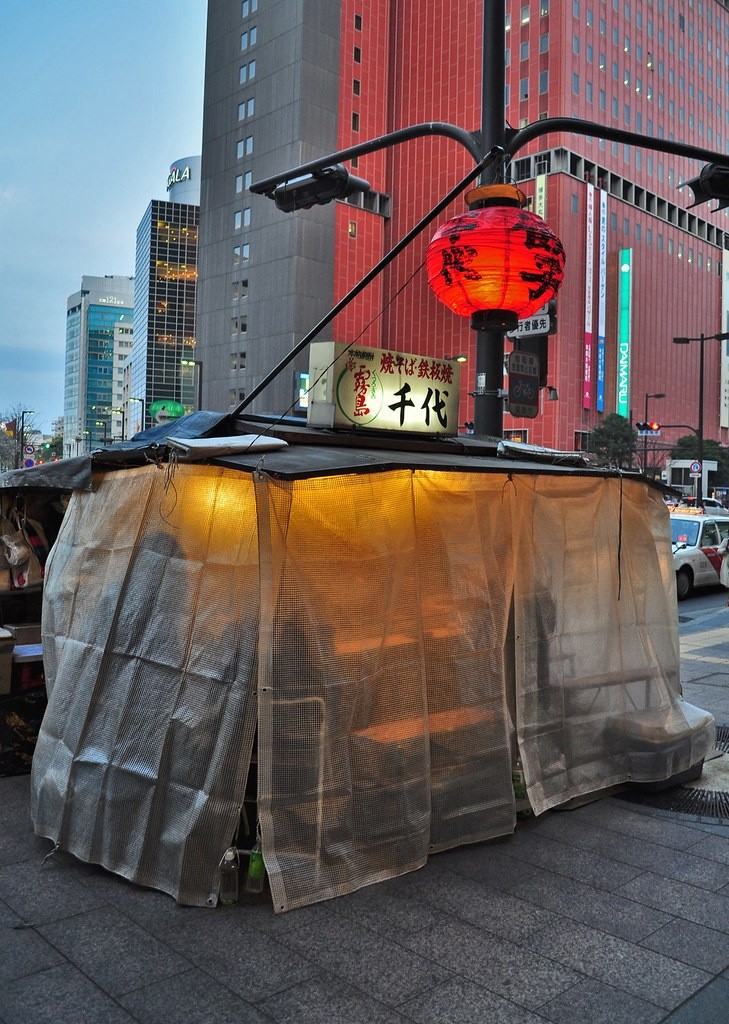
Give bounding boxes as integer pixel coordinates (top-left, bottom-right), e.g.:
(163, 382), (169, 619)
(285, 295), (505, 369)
(605, 702), (714, 793)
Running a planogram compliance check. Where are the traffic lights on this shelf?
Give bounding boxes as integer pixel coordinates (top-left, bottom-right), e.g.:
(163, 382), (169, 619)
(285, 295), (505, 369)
(636, 422), (661, 431)
(274, 163), (370, 213)
(676, 162), (729, 214)
(42, 443), (53, 448)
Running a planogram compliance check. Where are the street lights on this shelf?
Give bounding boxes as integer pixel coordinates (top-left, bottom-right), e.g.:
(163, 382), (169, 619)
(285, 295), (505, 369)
(17, 410), (34, 469)
(182, 359), (203, 411)
(128, 398), (144, 431)
(83, 430), (92, 452)
(644, 392), (666, 474)
(97, 421), (106, 446)
(112, 410), (124, 441)
(673, 332), (729, 506)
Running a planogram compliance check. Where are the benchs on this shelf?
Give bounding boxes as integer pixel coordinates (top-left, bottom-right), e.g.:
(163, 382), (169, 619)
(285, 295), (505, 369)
(348, 704), (503, 821)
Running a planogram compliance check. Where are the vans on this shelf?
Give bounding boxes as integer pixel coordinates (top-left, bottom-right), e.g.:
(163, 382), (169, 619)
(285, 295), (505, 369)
(679, 497), (729, 516)
(669, 514), (729, 600)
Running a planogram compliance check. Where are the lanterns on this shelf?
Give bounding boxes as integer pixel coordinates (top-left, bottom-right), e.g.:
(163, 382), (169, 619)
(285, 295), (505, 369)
(426, 183), (567, 331)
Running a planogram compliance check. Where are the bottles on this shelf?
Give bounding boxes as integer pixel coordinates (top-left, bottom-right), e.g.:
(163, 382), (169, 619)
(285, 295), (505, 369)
(218, 851), (239, 907)
(246, 836), (265, 894)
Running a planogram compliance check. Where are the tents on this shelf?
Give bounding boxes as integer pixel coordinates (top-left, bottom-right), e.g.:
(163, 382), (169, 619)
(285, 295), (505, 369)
(0, 410), (717, 915)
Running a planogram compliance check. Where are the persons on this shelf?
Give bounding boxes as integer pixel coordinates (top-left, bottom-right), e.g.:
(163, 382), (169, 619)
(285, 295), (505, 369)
(717, 528), (729, 607)
